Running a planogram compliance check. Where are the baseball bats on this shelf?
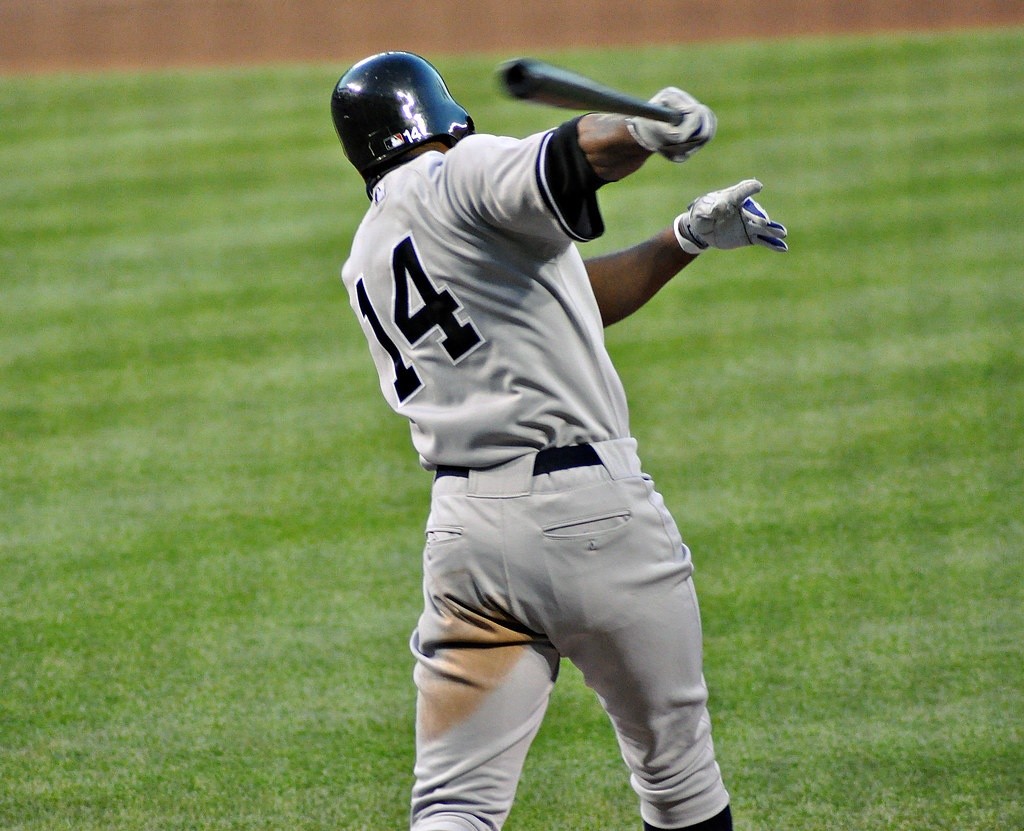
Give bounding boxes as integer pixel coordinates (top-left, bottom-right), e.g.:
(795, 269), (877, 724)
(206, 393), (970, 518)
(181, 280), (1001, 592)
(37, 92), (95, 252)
(503, 59), (685, 127)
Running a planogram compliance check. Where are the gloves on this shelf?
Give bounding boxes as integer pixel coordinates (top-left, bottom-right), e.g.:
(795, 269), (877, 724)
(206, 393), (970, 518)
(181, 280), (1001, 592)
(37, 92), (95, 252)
(625, 86), (717, 162)
(674, 179), (788, 255)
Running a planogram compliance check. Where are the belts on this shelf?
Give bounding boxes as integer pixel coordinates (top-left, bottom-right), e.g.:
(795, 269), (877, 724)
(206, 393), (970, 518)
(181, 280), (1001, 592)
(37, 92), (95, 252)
(435, 444), (602, 478)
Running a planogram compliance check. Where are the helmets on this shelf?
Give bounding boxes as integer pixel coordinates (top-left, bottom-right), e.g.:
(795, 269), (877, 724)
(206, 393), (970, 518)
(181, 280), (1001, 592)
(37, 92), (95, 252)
(331, 51), (475, 173)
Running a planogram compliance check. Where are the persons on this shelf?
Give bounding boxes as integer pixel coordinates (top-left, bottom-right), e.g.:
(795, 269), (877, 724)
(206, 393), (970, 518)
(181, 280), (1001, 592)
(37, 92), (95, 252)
(328, 52), (788, 831)
